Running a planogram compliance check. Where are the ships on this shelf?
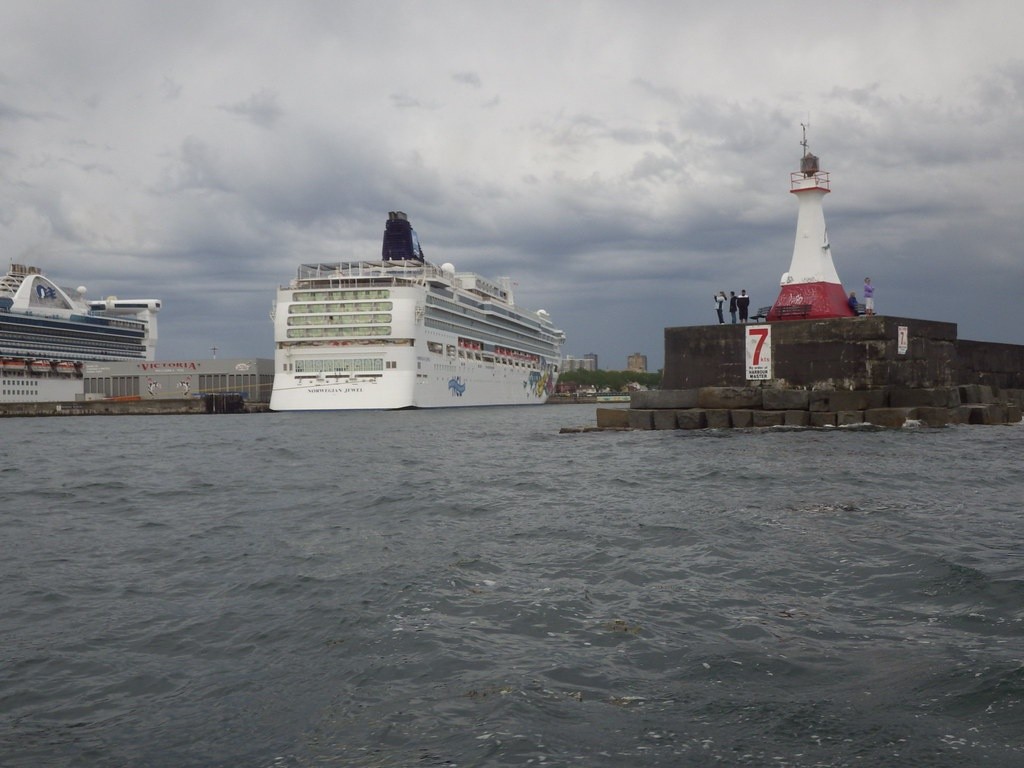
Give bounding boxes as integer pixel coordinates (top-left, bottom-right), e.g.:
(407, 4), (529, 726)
(0, 263), (162, 415)
(268, 210), (566, 412)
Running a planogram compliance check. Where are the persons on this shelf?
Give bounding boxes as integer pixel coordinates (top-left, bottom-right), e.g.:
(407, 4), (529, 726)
(848, 290), (859, 315)
(737, 290), (750, 323)
(863, 277), (876, 315)
(729, 291), (737, 323)
(714, 291), (727, 324)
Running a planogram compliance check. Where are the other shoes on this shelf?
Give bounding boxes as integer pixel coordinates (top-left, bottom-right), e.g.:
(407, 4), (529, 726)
(720, 323), (724, 325)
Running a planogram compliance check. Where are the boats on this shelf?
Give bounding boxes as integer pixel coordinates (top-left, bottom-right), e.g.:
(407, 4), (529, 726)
(57, 361), (76, 373)
(31, 359), (52, 373)
(1, 357), (26, 373)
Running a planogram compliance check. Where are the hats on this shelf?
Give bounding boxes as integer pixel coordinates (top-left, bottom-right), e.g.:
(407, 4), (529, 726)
(850, 290), (856, 296)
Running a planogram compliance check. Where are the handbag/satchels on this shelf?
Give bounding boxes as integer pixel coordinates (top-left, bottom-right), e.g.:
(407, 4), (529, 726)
(713, 301), (719, 309)
